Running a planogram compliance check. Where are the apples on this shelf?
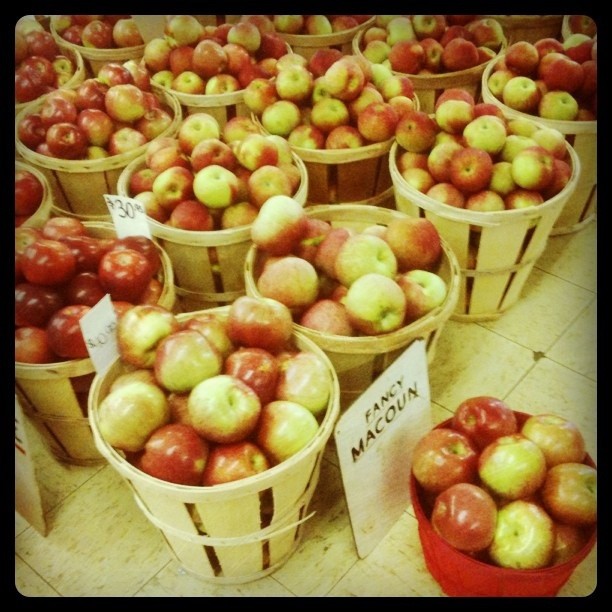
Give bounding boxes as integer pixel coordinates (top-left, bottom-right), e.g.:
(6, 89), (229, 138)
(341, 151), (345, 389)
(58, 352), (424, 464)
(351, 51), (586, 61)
(22, 60), (171, 161)
(242, 44), (415, 204)
(98, 292), (333, 570)
(362, 12), (502, 77)
(410, 394), (598, 571)
(15, 31), (73, 101)
(273, 14), (371, 40)
(395, 89), (572, 317)
(14, 216), (162, 418)
(143, 16), (289, 97)
(249, 195), (448, 339)
(128, 111), (302, 293)
(12, 167), (44, 226)
(487, 15), (596, 122)
(52, 14), (143, 49)
